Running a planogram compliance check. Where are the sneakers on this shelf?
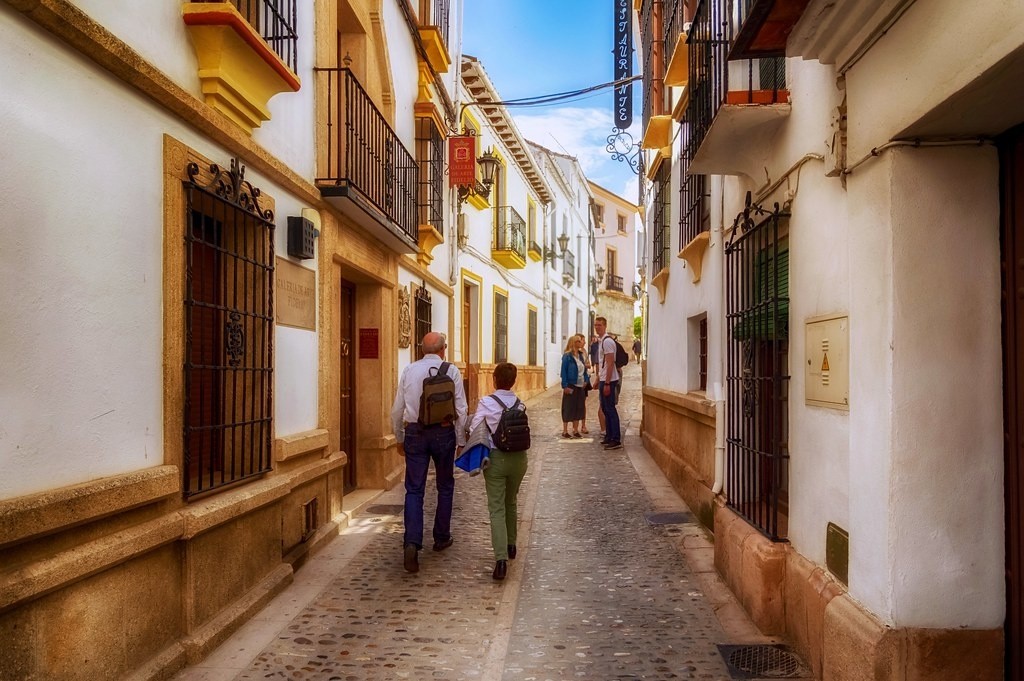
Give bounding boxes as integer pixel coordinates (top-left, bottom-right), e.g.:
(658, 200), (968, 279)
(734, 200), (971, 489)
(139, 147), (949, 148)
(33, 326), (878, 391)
(403, 543), (420, 572)
(562, 433), (583, 439)
(433, 536), (453, 551)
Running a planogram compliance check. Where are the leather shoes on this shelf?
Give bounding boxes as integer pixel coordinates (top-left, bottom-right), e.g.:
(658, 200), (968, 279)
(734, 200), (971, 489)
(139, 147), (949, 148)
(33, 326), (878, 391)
(507, 545), (516, 559)
(492, 560), (507, 580)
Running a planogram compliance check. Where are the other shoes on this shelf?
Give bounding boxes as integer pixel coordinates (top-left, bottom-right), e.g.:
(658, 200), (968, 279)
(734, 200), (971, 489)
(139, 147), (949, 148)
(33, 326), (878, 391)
(600, 440), (609, 444)
(604, 442), (621, 450)
(581, 428), (589, 434)
(597, 431), (606, 442)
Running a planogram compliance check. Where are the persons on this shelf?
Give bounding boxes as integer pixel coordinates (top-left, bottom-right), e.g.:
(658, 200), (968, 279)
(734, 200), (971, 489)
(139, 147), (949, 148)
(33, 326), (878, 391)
(392, 332), (468, 573)
(470, 362), (528, 580)
(560, 317), (642, 450)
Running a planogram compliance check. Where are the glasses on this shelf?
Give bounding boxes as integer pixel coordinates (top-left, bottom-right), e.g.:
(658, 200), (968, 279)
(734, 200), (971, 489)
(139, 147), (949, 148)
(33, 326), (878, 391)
(441, 344), (447, 350)
(575, 341), (581, 343)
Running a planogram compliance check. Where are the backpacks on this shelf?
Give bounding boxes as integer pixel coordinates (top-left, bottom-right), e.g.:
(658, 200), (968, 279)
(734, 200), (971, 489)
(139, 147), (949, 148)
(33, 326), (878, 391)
(602, 336), (628, 369)
(484, 395), (531, 453)
(418, 363), (459, 426)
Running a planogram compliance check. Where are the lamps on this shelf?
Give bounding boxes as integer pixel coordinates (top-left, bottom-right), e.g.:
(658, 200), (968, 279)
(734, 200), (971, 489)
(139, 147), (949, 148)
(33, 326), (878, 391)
(544, 234), (570, 265)
(591, 267), (606, 285)
(475, 147), (502, 198)
